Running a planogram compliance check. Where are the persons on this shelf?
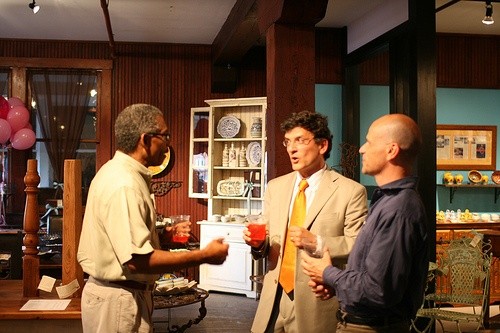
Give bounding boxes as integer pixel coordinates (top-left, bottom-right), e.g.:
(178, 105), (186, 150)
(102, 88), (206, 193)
(76, 104), (229, 333)
(300, 114), (429, 333)
(243, 110), (369, 333)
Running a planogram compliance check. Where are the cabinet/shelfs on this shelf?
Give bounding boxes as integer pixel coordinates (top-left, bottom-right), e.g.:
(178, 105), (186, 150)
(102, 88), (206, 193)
(435, 222), (500, 305)
(188, 96), (269, 298)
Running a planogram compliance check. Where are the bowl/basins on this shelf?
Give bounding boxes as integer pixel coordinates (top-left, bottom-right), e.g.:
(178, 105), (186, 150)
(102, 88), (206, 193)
(490, 170), (500, 185)
(467, 170), (482, 183)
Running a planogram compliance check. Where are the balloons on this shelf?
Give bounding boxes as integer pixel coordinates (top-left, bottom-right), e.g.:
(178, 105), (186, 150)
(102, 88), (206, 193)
(0, 95), (36, 150)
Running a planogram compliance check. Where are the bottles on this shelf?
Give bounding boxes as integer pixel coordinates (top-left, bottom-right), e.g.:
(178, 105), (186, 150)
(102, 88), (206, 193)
(193, 151), (208, 168)
(197, 171), (204, 193)
(222, 142), (229, 167)
(239, 142), (246, 166)
(229, 142), (236, 166)
(251, 117), (262, 138)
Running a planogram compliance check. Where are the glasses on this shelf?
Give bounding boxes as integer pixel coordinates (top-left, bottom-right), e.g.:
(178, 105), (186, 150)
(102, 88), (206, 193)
(281, 136), (315, 147)
(144, 132), (174, 140)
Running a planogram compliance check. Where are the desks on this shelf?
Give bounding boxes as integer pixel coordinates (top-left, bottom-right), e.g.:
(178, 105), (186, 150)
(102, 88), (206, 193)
(152, 287), (209, 333)
(0, 229), (88, 333)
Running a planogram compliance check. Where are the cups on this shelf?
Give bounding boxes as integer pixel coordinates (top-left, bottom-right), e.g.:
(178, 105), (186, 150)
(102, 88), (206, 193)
(168, 214), (191, 243)
(248, 216), (269, 241)
(302, 234), (325, 259)
(211, 213), (247, 223)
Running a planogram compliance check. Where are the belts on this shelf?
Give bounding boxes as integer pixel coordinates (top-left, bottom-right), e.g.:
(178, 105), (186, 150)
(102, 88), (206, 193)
(334, 307), (408, 326)
(108, 280), (157, 293)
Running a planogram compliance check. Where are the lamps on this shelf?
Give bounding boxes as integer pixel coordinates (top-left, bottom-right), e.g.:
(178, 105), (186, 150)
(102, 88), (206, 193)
(482, 2), (494, 25)
(29, 0), (39, 13)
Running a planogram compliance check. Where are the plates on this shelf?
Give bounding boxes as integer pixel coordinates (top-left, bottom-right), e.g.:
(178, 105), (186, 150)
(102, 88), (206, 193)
(217, 116), (241, 138)
(217, 180), (245, 196)
(246, 142), (262, 167)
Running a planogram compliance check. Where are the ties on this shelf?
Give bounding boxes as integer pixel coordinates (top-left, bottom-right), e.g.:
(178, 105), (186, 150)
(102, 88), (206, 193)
(279, 179), (309, 293)
